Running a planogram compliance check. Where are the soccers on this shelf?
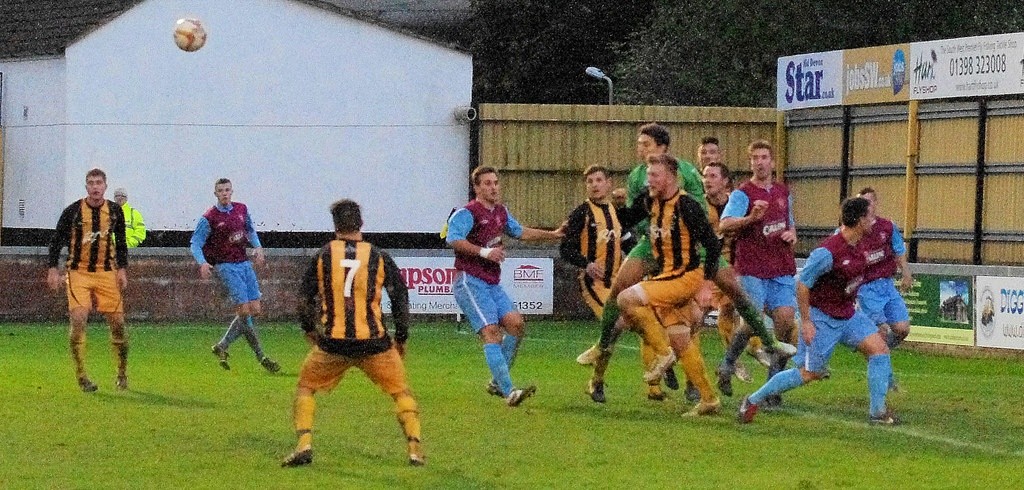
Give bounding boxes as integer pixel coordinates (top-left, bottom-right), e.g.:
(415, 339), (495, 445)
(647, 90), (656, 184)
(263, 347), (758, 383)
(173, 19), (206, 52)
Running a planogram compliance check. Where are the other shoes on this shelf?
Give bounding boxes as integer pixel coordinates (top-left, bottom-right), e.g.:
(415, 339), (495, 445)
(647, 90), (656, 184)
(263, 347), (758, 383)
(282, 446), (312, 469)
(78, 377), (99, 393)
(117, 373), (127, 389)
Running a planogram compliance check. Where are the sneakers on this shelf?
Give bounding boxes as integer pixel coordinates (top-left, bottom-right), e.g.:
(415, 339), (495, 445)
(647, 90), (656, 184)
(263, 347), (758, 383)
(715, 366), (731, 396)
(649, 390), (669, 402)
(681, 398), (723, 419)
(748, 347), (771, 367)
(644, 346), (675, 382)
(256, 355), (282, 372)
(684, 381), (698, 399)
(211, 342), (231, 371)
(585, 378), (608, 402)
(732, 362), (755, 384)
(506, 386), (536, 405)
(869, 413), (911, 428)
(737, 394), (757, 423)
(762, 401), (795, 417)
(577, 342), (614, 364)
(487, 381), (505, 399)
(662, 366), (678, 389)
(765, 341), (796, 357)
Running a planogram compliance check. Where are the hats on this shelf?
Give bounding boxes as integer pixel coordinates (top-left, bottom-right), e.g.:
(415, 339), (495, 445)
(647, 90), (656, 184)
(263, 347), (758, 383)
(113, 188), (127, 202)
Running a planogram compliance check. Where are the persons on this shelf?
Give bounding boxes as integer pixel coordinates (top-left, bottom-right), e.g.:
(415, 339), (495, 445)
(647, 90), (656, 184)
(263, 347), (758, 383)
(733, 197), (908, 426)
(447, 165), (566, 404)
(281, 199), (428, 466)
(190, 178), (282, 374)
(46, 169), (130, 391)
(558, 120), (802, 422)
(113, 186), (146, 248)
(833, 187), (912, 348)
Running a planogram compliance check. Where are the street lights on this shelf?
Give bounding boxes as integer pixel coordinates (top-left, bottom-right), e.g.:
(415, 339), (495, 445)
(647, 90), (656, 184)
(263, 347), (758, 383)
(585, 67), (614, 107)
